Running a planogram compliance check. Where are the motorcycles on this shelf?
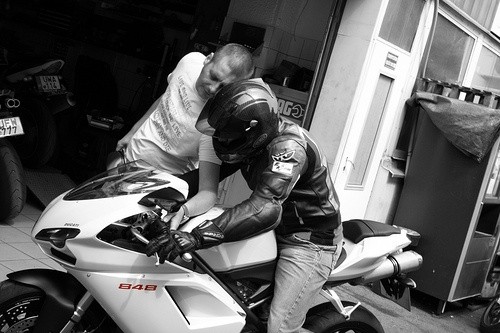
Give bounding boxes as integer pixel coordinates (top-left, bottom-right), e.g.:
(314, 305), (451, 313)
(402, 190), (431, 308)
(0, 156), (424, 332)
(480, 296), (500, 332)
(0, 59), (68, 222)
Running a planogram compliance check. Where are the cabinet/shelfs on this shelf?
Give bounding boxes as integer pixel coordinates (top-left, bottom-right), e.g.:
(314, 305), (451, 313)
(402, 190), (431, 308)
(395, 90), (500, 313)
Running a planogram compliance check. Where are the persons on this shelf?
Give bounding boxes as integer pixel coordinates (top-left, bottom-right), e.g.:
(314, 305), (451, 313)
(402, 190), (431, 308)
(5, 46), (71, 99)
(105, 42), (255, 231)
(145, 77), (344, 333)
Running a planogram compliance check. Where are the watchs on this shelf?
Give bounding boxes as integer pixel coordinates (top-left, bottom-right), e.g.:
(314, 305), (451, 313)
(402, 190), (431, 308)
(180, 204), (189, 225)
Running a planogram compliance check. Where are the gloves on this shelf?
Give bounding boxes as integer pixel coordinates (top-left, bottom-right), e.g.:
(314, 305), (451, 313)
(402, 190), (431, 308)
(146, 220), (224, 264)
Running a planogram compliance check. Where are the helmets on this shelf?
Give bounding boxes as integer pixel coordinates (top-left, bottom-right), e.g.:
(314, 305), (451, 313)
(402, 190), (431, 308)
(196, 78), (278, 165)
